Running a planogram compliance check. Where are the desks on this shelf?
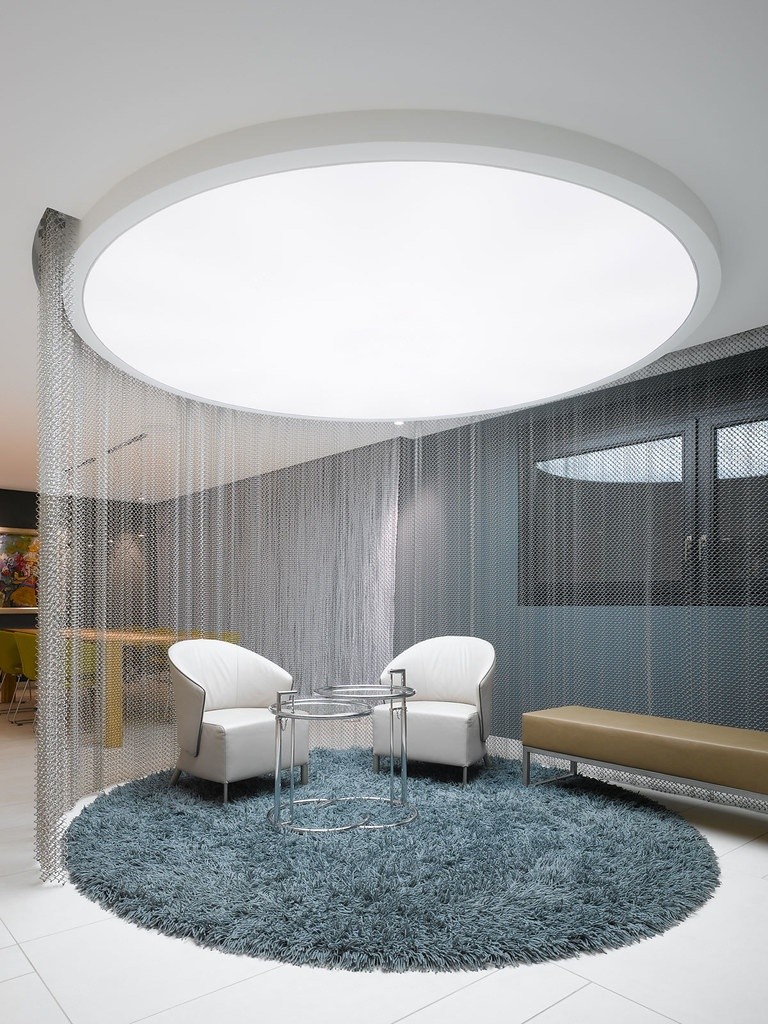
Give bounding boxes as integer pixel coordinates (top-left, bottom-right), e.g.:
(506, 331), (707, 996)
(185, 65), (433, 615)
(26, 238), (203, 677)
(270, 690), (373, 832)
(6, 629), (231, 747)
(320, 668), (416, 828)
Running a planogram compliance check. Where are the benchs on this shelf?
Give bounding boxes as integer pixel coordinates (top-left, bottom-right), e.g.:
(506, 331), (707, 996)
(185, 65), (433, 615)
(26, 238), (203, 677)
(522, 705), (768, 816)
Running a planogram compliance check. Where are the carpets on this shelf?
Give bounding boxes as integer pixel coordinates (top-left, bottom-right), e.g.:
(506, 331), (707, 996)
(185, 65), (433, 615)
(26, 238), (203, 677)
(60, 744), (722, 973)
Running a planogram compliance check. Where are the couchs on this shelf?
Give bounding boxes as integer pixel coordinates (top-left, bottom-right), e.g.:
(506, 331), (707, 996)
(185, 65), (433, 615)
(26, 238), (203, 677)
(168, 638), (307, 805)
(371, 634), (495, 782)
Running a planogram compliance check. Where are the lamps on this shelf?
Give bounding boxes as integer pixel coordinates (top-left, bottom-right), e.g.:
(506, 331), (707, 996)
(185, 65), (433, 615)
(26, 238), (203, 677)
(63, 112), (720, 423)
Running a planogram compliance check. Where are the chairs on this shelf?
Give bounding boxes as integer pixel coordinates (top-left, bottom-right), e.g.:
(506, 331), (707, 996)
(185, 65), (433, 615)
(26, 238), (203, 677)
(0, 630), (100, 738)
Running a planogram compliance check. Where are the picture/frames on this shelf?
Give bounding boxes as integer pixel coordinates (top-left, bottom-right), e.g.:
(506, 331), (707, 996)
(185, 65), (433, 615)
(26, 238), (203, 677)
(0, 527), (39, 614)
